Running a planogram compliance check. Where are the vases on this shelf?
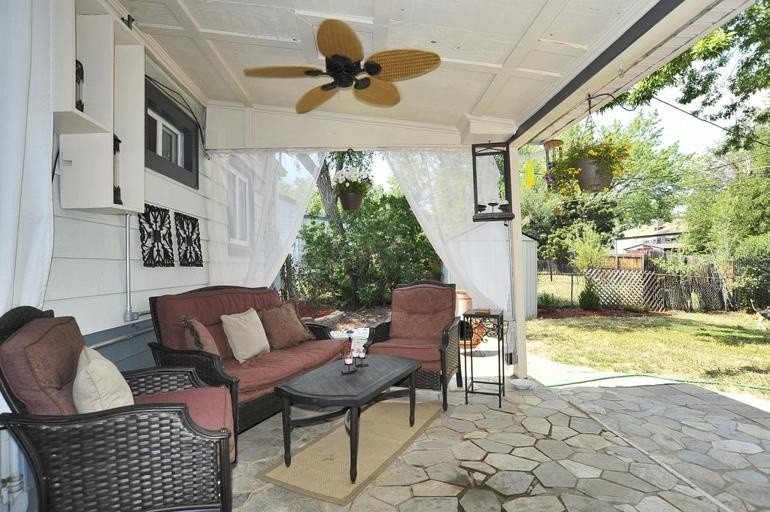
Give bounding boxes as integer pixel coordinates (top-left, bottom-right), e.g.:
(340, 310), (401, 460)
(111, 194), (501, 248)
(574, 159), (612, 193)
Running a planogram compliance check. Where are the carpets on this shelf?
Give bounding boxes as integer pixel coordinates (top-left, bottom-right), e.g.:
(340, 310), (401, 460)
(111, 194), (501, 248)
(255, 402), (443, 506)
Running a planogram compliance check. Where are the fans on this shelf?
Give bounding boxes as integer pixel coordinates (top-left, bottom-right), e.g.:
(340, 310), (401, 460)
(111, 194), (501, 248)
(243, 18), (441, 113)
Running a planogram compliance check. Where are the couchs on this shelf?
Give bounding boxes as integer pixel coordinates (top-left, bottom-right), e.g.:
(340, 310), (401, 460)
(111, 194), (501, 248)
(146, 286), (353, 434)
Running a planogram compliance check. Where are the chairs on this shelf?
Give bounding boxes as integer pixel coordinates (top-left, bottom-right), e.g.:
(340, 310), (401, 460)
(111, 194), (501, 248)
(0, 306), (235, 512)
(362, 279), (461, 411)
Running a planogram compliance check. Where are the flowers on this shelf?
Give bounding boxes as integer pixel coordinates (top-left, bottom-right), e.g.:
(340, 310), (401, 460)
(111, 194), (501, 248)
(541, 142), (640, 193)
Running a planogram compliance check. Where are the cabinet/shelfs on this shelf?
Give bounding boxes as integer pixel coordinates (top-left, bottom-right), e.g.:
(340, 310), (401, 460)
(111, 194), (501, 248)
(50, 1), (146, 216)
(463, 308), (507, 408)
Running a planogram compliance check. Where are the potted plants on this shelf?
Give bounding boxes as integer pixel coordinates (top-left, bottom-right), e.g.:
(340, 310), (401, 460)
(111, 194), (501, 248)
(332, 177), (374, 212)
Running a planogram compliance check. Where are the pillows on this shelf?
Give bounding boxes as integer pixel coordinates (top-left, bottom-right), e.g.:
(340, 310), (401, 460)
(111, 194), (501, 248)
(183, 315), (219, 355)
(221, 307), (270, 364)
(73, 345), (134, 414)
(258, 298), (313, 350)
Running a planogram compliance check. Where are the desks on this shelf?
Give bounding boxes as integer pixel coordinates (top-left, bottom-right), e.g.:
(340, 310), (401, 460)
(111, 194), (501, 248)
(274, 353), (422, 483)
